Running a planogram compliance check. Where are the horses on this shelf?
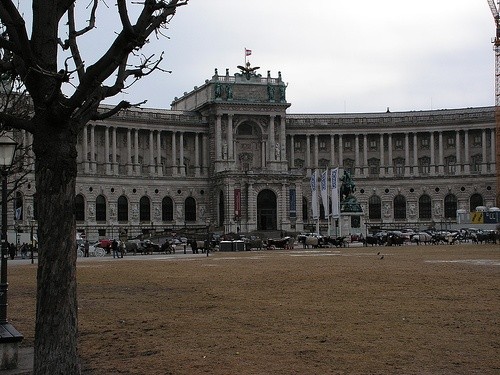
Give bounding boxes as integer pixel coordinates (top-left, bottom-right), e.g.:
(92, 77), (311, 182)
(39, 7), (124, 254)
(298, 230), (484, 249)
(187, 238), (208, 254)
(95, 240), (111, 255)
(125, 241), (138, 255)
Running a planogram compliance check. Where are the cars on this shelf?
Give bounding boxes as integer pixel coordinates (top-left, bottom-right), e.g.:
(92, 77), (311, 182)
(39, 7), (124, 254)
(351, 232), (364, 243)
(296, 233), (325, 243)
(93, 238), (163, 252)
(366, 228), (496, 246)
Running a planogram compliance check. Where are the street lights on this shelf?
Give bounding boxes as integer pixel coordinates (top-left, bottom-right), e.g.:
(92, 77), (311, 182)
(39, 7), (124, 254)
(29, 218), (35, 264)
(0, 135), (21, 325)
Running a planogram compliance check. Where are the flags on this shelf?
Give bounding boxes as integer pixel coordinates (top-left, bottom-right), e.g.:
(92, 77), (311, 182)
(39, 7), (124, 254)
(245, 50), (251, 56)
(321, 170), (329, 219)
(331, 167), (340, 218)
(311, 171), (320, 220)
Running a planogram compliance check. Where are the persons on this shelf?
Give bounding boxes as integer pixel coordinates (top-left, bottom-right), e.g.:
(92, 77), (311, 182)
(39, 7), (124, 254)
(112, 239), (120, 258)
(164, 239), (172, 254)
(119, 239), (125, 257)
(132, 239), (154, 255)
(107, 239), (111, 254)
(84, 240), (89, 257)
(8, 242), (30, 260)
(339, 170), (347, 192)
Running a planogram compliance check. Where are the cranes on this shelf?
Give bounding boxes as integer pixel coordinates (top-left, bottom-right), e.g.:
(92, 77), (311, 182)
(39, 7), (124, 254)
(486, 0), (500, 130)
